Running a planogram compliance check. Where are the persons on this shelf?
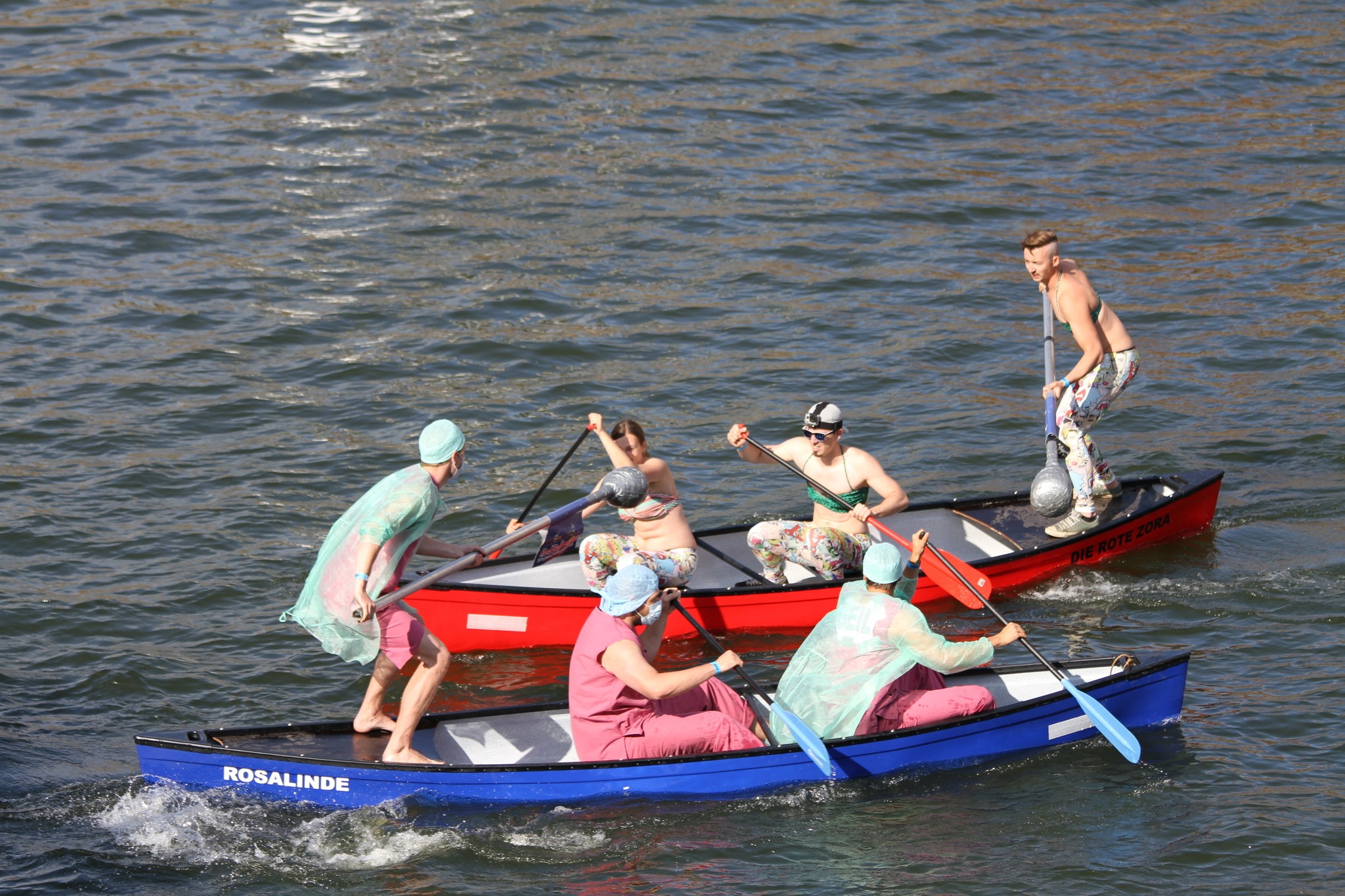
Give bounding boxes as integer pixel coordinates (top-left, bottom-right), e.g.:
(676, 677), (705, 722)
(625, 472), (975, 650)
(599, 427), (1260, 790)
(279, 419), (488, 765)
(1020, 230), (1140, 539)
(727, 401), (910, 588)
(763, 529), (1026, 747)
(506, 413), (698, 596)
(568, 563), (766, 762)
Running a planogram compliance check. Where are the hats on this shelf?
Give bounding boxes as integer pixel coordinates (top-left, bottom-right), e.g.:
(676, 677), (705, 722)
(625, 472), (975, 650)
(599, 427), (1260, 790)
(808, 402), (849, 434)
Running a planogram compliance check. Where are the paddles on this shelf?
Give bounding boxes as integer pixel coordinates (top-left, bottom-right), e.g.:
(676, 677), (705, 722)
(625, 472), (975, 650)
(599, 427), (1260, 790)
(489, 420), (605, 559)
(733, 425), (992, 609)
(918, 531), (1142, 765)
(668, 585), (831, 779)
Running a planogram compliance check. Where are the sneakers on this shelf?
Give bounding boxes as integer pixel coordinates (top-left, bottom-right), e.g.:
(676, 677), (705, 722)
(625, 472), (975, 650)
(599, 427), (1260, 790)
(1045, 508), (1100, 538)
(1073, 481), (1122, 499)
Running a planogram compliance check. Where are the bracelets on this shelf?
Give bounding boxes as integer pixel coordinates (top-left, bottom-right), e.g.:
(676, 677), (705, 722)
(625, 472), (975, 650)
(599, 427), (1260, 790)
(906, 557), (921, 570)
(1060, 378), (1070, 389)
(354, 573), (370, 582)
(710, 662), (721, 677)
(737, 441), (749, 450)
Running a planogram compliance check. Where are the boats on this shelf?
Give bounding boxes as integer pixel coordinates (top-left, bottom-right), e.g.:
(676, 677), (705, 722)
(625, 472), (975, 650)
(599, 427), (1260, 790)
(396, 464), (1226, 656)
(133, 646), (1192, 806)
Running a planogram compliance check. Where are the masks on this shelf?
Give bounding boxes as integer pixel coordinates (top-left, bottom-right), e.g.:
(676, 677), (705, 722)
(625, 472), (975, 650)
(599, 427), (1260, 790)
(635, 599), (662, 625)
(449, 452), (465, 479)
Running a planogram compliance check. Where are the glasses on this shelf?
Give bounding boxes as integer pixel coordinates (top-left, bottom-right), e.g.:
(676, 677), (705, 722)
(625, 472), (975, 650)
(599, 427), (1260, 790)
(802, 426), (834, 442)
